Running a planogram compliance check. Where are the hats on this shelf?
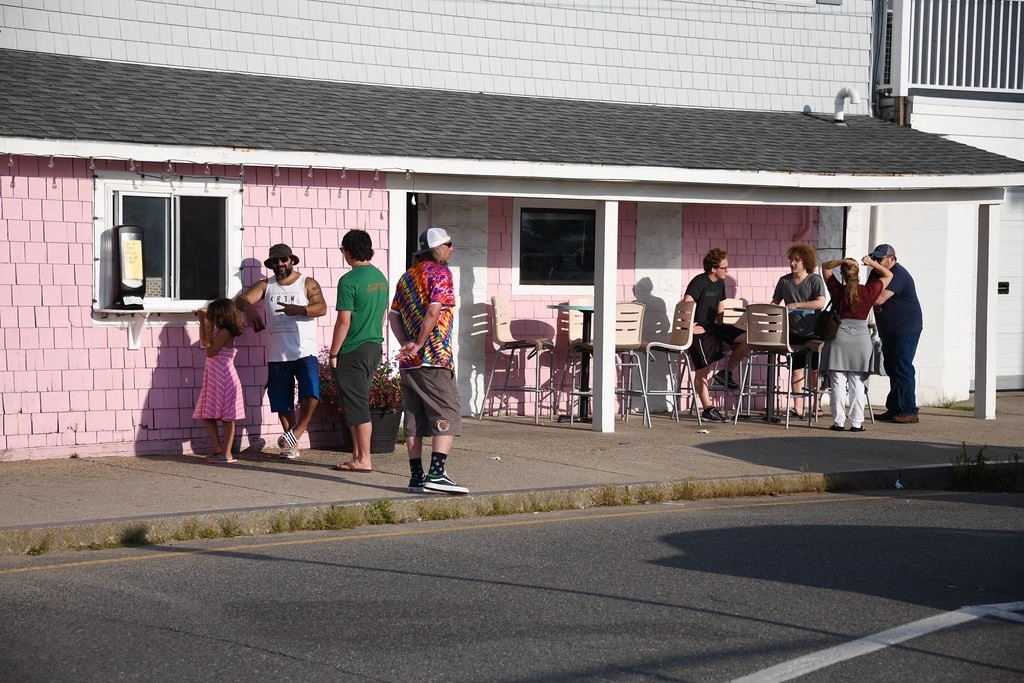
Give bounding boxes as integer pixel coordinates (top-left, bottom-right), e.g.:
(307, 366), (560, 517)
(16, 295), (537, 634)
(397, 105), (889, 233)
(263, 244), (299, 270)
(867, 243), (895, 258)
(413, 227), (451, 254)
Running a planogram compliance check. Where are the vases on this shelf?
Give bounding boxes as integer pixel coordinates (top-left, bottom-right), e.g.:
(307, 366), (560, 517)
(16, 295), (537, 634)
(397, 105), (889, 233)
(343, 408), (402, 453)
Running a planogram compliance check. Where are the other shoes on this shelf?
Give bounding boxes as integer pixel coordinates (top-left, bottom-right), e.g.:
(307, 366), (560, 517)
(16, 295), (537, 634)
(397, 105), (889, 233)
(829, 423), (844, 431)
(873, 410), (892, 420)
(892, 412), (919, 423)
(850, 424), (866, 432)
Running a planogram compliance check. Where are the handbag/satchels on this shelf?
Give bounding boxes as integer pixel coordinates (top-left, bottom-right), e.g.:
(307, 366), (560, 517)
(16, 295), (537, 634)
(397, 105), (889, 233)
(813, 308), (841, 340)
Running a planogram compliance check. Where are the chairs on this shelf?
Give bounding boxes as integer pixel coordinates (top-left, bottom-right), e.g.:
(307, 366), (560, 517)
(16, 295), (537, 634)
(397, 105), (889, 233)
(477, 295), (556, 424)
(708, 298), (782, 419)
(616, 302), (652, 430)
(624, 301), (703, 426)
(555, 297), (593, 425)
(802, 340), (875, 423)
(734, 302), (813, 431)
(668, 349), (730, 421)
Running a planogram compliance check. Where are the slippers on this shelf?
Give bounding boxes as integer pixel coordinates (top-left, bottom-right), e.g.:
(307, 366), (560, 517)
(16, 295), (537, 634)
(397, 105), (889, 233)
(212, 454), (238, 464)
(278, 423), (303, 450)
(781, 409), (804, 419)
(812, 407), (824, 418)
(332, 461), (372, 473)
(278, 446), (300, 459)
(204, 452), (213, 458)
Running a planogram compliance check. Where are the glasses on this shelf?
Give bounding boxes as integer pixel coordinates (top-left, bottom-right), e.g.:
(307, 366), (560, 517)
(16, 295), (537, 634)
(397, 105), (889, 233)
(787, 257), (803, 263)
(871, 257), (883, 263)
(269, 256), (289, 265)
(442, 241), (452, 248)
(339, 247), (344, 253)
(717, 265), (729, 272)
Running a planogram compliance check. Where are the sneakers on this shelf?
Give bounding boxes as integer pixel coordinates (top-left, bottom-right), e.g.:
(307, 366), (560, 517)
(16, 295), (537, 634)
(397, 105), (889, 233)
(407, 473), (429, 493)
(715, 370), (738, 389)
(424, 470), (469, 494)
(701, 407), (731, 423)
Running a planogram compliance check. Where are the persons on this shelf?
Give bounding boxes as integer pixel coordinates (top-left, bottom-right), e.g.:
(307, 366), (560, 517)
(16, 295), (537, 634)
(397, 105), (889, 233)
(330, 230), (389, 471)
(682, 248), (748, 422)
(865, 244), (922, 423)
(192, 298), (246, 464)
(769, 242), (826, 419)
(238, 244), (327, 458)
(822, 256), (893, 431)
(389, 227), (471, 494)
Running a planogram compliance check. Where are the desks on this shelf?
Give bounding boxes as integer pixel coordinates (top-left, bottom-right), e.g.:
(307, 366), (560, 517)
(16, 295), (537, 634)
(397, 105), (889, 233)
(733, 306), (815, 425)
(546, 305), (593, 424)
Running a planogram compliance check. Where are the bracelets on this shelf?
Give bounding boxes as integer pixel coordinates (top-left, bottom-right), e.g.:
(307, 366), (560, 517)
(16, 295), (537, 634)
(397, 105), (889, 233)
(330, 354), (337, 359)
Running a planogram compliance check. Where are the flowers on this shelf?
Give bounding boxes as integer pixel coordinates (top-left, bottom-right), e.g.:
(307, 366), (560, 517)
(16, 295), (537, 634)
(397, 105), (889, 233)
(318, 343), (404, 414)
